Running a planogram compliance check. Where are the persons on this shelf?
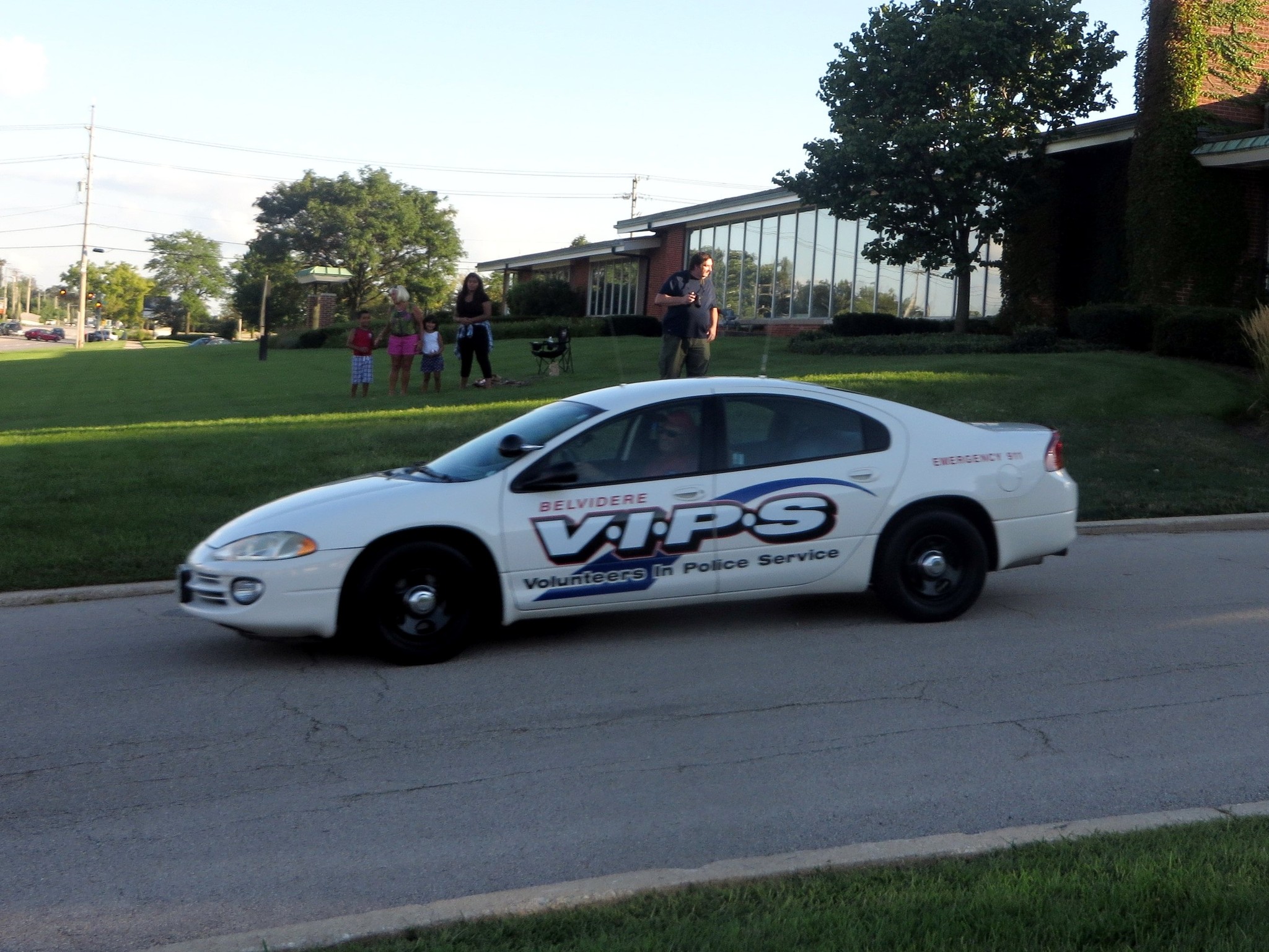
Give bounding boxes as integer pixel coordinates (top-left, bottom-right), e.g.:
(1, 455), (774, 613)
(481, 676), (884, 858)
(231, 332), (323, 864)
(374, 284), (423, 402)
(654, 252), (718, 379)
(346, 311), (379, 400)
(572, 408), (698, 485)
(417, 315), (445, 394)
(451, 273), (493, 389)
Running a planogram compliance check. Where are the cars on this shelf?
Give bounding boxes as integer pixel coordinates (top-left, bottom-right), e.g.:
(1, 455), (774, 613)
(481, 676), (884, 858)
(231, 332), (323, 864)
(24, 328), (66, 342)
(85, 329), (119, 343)
(45, 319), (57, 325)
(190, 336), (242, 346)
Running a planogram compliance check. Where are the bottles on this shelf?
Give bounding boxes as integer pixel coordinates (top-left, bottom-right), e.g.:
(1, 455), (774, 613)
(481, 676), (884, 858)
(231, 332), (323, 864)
(548, 337), (553, 342)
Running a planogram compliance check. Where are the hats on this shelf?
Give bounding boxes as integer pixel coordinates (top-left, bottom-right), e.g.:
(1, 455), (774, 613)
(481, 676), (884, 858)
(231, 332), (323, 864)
(664, 409), (693, 429)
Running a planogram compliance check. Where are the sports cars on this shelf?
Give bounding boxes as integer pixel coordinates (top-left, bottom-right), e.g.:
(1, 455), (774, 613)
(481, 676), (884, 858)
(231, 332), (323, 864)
(176, 374), (1078, 665)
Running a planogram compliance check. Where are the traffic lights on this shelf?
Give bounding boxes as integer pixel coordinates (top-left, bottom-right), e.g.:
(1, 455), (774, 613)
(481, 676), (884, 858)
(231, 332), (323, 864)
(96, 302), (101, 307)
(88, 292), (102, 298)
(46, 287), (66, 295)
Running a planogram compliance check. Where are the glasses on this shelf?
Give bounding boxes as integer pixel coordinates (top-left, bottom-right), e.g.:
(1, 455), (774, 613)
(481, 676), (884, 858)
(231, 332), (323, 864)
(656, 425), (688, 438)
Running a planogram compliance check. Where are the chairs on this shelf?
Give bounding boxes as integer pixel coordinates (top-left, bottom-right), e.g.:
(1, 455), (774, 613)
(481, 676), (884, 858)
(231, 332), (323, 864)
(528, 325), (574, 374)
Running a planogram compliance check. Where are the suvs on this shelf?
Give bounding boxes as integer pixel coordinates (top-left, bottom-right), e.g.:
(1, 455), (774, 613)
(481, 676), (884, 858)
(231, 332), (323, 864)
(0, 322), (22, 331)
